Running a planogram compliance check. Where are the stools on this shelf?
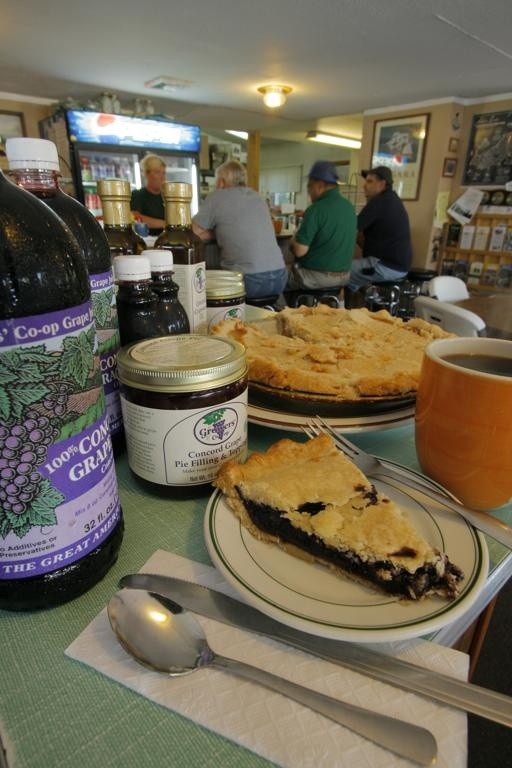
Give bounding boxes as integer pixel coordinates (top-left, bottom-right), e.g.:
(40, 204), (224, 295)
(294, 287), (342, 308)
(400, 268), (437, 316)
(246, 294), (279, 310)
(366, 278), (404, 316)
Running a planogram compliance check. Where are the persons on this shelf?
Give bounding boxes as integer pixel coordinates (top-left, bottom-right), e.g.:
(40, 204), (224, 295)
(129, 156), (169, 233)
(275, 161), (357, 308)
(356, 166), (413, 300)
(192, 160), (288, 297)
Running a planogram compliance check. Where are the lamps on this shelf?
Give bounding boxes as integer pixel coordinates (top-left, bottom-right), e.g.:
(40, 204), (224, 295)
(257, 84), (293, 108)
(305, 130), (362, 150)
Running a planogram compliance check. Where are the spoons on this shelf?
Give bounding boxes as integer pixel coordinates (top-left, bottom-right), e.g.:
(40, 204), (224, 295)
(106, 587), (440, 765)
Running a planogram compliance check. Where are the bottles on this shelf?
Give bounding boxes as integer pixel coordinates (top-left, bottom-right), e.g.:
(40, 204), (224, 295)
(152, 179), (209, 333)
(111, 250), (161, 346)
(1, 169), (127, 618)
(8, 136), (128, 466)
(142, 246), (190, 332)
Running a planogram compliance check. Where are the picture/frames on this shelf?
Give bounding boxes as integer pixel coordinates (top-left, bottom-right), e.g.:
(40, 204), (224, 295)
(0, 110), (27, 156)
(442, 157), (458, 178)
(370, 113), (430, 201)
(448, 138), (460, 152)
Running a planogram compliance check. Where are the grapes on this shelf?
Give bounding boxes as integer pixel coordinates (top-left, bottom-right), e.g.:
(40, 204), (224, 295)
(0, 373), (67, 515)
(211, 419), (229, 442)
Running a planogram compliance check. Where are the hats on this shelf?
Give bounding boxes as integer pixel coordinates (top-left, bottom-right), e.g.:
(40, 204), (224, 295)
(306, 161), (337, 183)
(360, 167), (393, 187)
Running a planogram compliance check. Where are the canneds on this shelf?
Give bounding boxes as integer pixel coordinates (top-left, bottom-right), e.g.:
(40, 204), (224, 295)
(288, 215), (297, 233)
(205, 269), (247, 334)
(115, 331), (250, 500)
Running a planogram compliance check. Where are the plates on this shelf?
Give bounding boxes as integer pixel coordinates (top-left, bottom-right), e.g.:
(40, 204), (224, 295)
(201, 455), (490, 645)
(216, 402), (450, 436)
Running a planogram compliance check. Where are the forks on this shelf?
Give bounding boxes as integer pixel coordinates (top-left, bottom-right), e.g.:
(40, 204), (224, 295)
(302, 415), (512, 549)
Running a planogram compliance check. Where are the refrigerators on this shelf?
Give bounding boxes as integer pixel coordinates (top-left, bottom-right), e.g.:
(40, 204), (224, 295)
(36, 112), (201, 235)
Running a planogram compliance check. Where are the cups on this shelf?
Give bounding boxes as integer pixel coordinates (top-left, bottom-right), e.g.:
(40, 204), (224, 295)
(415, 338), (512, 507)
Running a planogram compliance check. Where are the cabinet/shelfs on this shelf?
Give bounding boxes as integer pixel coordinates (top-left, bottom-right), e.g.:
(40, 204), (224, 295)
(435, 214), (511, 293)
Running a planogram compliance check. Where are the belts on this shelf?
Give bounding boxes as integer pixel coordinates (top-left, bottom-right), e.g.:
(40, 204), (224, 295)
(323, 271), (349, 276)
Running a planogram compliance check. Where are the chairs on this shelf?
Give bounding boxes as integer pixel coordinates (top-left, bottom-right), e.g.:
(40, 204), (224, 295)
(428, 275), (470, 304)
(415, 295), (488, 339)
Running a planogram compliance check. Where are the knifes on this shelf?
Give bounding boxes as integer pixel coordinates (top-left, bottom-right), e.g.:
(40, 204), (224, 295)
(117, 572), (512, 729)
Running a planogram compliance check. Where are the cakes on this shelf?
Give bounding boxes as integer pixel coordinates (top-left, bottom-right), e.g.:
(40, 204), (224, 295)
(214, 302), (460, 398)
(212, 430), (466, 605)
